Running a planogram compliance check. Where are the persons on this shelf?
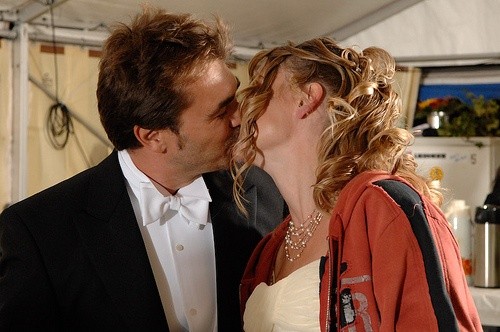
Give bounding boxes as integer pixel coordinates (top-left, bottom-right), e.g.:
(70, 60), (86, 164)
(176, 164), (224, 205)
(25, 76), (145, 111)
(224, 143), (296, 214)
(0, 5), (289, 331)
(227, 37), (483, 332)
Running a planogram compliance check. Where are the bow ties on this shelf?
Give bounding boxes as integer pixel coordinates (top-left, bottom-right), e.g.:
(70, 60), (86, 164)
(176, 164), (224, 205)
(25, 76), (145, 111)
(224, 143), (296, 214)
(138, 179), (213, 226)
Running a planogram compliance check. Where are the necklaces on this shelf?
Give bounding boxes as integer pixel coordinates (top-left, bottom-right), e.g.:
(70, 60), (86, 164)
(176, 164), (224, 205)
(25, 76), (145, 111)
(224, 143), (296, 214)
(284, 207), (327, 263)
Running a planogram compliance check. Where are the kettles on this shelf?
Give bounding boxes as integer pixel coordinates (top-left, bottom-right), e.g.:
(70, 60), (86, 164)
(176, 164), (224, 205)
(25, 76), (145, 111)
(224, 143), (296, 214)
(443, 199), (475, 287)
(424, 179), (443, 209)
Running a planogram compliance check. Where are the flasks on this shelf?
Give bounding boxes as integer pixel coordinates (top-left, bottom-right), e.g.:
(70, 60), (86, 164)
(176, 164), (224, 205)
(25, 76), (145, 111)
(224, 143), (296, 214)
(472, 204), (500, 289)
